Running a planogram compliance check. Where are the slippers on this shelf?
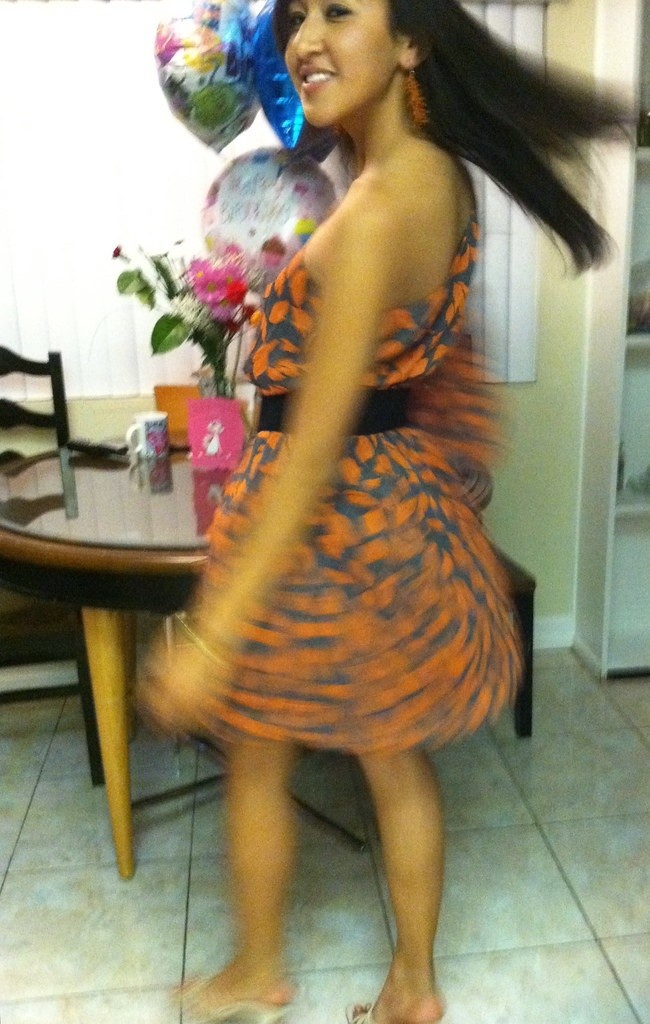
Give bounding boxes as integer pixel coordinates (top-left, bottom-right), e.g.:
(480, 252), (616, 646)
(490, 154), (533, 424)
(171, 978), (292, 1024)
(345, 1003), (376, 1024)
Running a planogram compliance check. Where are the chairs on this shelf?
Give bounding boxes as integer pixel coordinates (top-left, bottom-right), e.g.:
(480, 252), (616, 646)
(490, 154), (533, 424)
(1, 344), (105, 789)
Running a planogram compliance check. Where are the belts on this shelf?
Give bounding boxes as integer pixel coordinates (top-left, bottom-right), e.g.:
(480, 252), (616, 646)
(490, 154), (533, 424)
(257, 384), (411, 436)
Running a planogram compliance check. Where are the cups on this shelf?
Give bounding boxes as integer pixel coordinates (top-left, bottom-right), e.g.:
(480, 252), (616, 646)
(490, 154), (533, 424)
(125, 411), (168, 459)
(125, 456), (174, 497)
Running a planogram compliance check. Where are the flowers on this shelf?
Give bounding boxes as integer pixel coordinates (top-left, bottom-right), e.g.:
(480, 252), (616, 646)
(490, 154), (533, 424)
(113, 235), (286, 399)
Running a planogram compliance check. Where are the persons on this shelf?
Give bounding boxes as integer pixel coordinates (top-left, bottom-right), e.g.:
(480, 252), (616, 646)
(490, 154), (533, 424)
(139, 0), (633, 1024)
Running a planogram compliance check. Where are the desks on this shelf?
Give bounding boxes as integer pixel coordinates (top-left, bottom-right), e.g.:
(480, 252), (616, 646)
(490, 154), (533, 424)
(1, 450), (369, 883)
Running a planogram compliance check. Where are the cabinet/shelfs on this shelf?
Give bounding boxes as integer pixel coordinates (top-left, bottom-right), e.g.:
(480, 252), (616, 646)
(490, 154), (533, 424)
(571, 0), (650, 681)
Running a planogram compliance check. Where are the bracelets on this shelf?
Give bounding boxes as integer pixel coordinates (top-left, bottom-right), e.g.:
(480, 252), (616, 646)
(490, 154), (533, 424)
(180, 613), (234, 672)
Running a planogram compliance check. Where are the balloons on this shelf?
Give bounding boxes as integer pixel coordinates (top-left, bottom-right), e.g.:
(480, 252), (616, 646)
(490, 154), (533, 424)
(251, 0), (343, 160)
(202, 145), (335, 289)
(156, 0), (259, 157)
